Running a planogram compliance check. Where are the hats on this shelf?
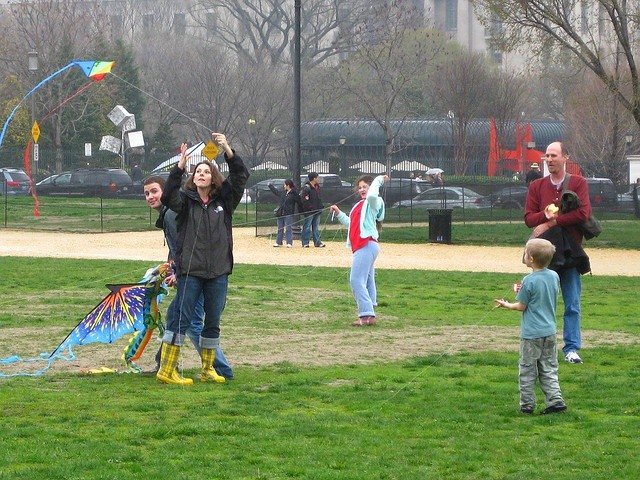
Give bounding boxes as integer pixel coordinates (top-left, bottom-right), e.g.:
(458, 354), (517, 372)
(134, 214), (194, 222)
(531, 163), (539, 168)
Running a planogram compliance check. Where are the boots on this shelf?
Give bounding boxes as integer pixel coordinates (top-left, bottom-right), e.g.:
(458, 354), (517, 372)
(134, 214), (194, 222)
(155, 342), (193, 386)
(198, 349), (226, 384)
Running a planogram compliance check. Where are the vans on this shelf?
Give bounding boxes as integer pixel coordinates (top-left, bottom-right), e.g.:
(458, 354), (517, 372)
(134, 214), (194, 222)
(299, 173), (341, 190)
(586, 177), (622, 212)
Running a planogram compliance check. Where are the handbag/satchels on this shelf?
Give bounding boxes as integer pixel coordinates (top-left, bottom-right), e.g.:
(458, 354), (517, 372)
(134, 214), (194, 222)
(273, 193), (291, 217)
(522, 172), (577, 273)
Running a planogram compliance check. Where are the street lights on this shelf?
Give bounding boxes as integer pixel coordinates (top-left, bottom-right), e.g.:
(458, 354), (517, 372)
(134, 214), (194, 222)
(28, 51), (38, 188)
(618, 130), (637, 208)
(339, 136), (346, 177)
(448, 109), (455, 143)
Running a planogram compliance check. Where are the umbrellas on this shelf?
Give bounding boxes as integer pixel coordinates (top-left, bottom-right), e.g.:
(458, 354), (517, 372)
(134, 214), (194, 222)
(425, 168), (445, 174)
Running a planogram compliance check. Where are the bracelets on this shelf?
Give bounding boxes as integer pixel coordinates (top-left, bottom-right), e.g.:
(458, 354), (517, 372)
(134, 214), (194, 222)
(545, 220), (554, 229)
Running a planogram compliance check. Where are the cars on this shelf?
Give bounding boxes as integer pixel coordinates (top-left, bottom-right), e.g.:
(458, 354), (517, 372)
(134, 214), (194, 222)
(490, 187), (528, 208)
(248, 179), (296, 203)
(391, 187), (492, 211)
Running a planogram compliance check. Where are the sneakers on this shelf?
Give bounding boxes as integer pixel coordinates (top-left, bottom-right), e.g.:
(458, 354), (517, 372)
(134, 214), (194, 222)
(352, 316), (374, 326)
(520, 406), (533, 415)
(315, 244), (325, 247)
(273, 244), (282, 247)
(286, 244), (292, 248)
(541, 401), (567, 415)
(564, 352), (582, 364)
(302, 244), (309, 247)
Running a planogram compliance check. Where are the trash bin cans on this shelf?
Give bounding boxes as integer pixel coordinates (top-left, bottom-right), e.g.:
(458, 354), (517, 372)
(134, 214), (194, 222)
(427, 208), (453, 245)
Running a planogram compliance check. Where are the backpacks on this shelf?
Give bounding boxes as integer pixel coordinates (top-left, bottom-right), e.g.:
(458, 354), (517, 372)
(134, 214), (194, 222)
(297, 184), (311, 213)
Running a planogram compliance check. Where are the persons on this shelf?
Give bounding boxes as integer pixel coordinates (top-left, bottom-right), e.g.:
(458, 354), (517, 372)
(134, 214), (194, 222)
(144, 176), (234, 379)
(630, 178), (640, 218)
(408, 171), (415, 178)
(132, 163), (142, 181)
(415, 173), (423, 193)
(524, 141), (593, 363)
(156, 132), (250, 384)
(300, 172), (326, 247)
(493, 238), (568, 415)
(526, 163), (544, 183)
(267, 180), (310, 248)
(426, 173), (444, 186)
(330, 174), (390, 326)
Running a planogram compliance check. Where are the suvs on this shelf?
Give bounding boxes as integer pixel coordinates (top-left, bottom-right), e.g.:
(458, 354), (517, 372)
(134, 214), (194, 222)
(33, 167), (133, 199)
(385, 179), (432, 204)
(0, 167), (32, 195)
(132, 172), (187, 194)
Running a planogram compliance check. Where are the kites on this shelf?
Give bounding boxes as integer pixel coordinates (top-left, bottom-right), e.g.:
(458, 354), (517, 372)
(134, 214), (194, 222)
(89, 264), (166, 374)
(247, 119), (256, 125)
(1, 284), (169, 377)
(0, 60), (117, 218)
